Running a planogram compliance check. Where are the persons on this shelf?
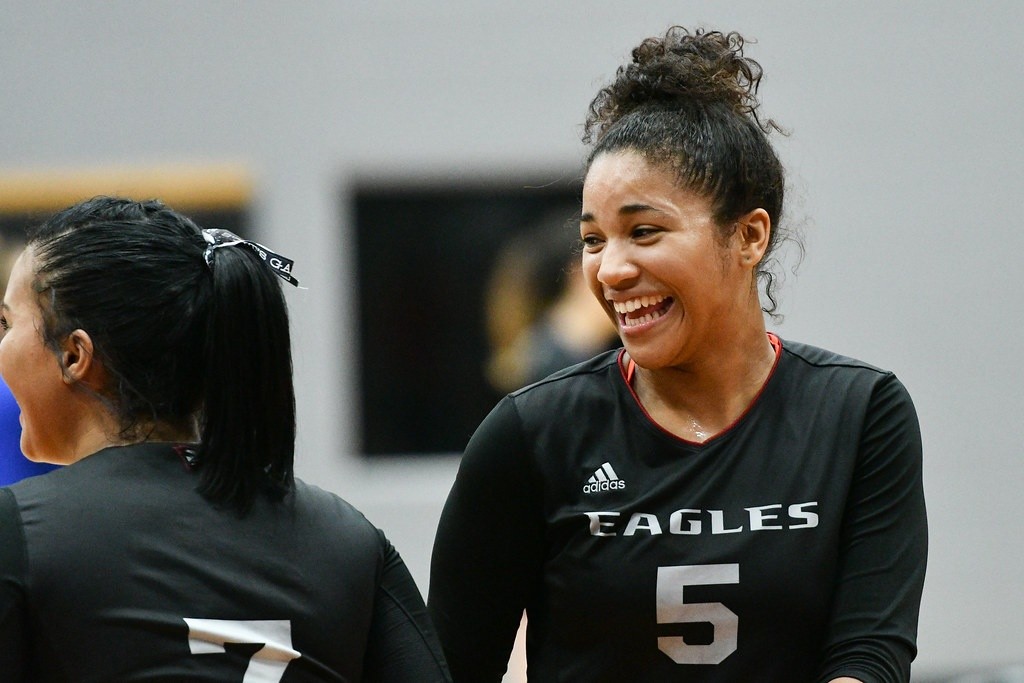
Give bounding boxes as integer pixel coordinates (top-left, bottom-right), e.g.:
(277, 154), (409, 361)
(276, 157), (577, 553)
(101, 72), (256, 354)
(0, 196), (456, 683)
(427, 26), (928, 683)
